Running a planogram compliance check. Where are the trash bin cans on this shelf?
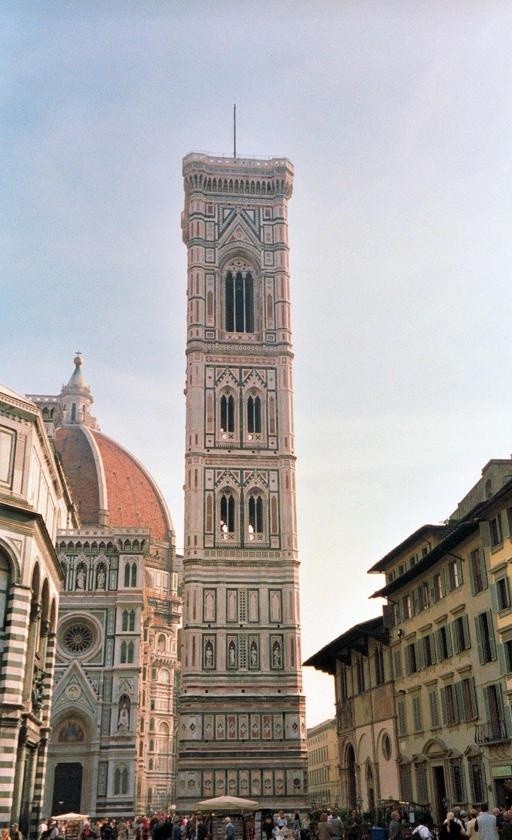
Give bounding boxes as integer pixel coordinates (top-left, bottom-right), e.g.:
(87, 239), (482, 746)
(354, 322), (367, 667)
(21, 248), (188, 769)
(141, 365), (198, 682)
(369, 827), (386, 840)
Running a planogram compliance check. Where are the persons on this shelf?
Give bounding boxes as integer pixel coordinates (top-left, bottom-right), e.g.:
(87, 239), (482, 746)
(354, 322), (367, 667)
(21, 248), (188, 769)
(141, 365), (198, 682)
(274, 646), (279, 667)
(37, 817), (67, 840)
(206, 645), (214, 665)
(229, 646), (236, 666)
(251, 646), (258, 665)
(132, 814), (256, 840)
(80, 817), (132, 840)
(1, 823), (22, 840)
(263, 802), (512, 840)
(116, 702), (130, 733)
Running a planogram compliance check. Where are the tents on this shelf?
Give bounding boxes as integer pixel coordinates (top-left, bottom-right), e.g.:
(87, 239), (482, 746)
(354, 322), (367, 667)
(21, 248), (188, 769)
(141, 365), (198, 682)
(51, 812), (89, 822)
(193, 795), (260, 814)
